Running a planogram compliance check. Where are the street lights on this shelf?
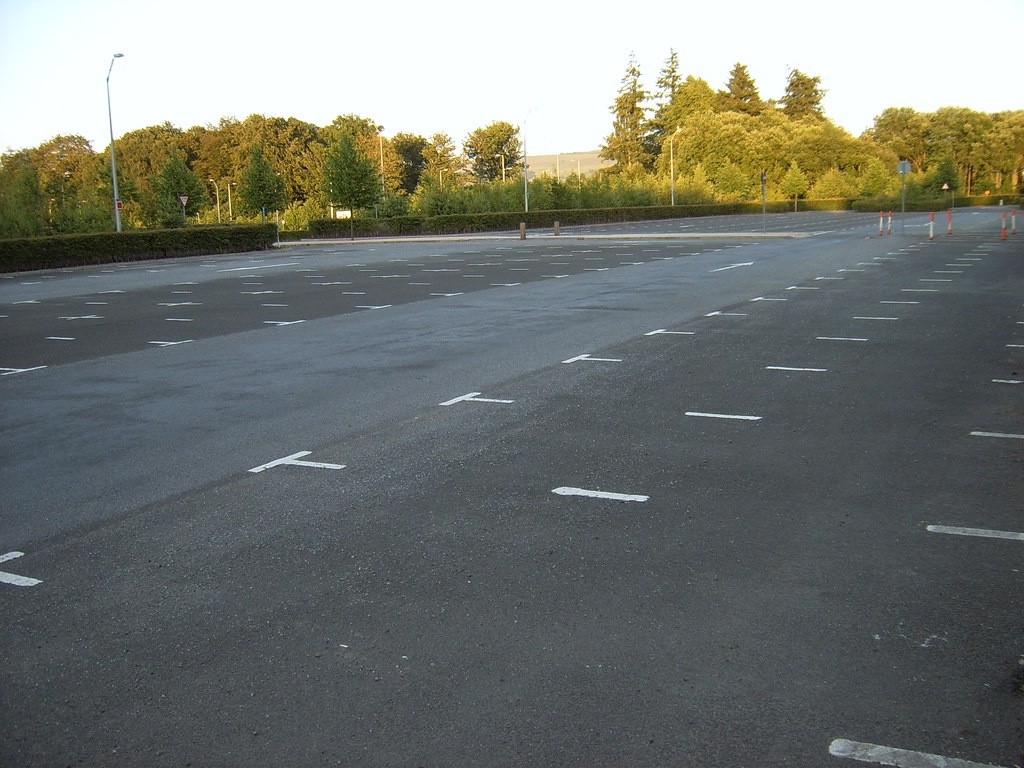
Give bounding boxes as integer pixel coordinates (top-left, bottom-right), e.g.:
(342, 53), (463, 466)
(106, 52), (125, 234)
(671, 127), (683, 205)
